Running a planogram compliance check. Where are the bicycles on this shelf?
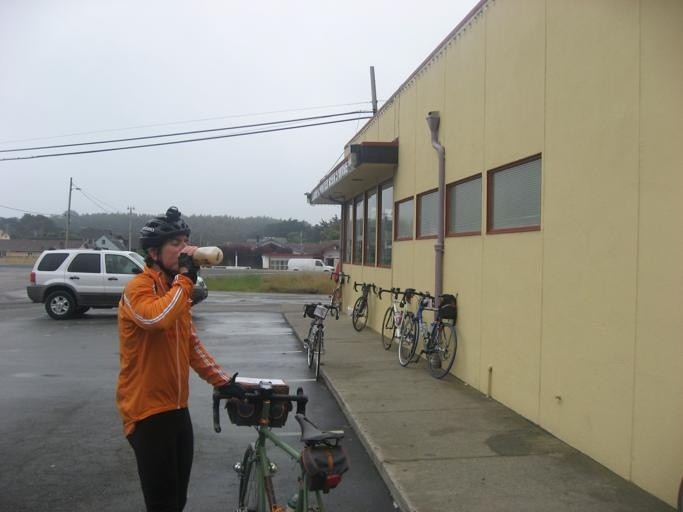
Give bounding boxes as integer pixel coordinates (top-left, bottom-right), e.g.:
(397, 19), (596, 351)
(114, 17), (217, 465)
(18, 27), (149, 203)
(329, 272), (351, 316)
(303, 302), (339, 382)
(378, 288), (457, 379)
(352, 281), (382, 332)
(212, 380), (345, 511)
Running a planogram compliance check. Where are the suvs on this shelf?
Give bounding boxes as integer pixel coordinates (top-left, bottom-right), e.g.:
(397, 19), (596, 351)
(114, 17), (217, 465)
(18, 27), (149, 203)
(27, 246), (208, 320)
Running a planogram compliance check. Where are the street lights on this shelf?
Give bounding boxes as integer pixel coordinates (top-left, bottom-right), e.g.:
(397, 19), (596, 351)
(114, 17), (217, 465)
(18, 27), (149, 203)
(127, 202), (134, 251)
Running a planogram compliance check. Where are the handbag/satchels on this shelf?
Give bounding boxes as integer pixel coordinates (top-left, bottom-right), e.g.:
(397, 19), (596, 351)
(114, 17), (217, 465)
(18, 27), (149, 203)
(439, 294), (457, 319)
(307, 305), (329, 319)
(302, 445), (347, 491)
(226, 397), (288, 426)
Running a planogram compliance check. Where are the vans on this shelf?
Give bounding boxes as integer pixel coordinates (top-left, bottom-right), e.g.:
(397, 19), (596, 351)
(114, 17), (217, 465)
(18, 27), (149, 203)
(288, 258), (335, 272)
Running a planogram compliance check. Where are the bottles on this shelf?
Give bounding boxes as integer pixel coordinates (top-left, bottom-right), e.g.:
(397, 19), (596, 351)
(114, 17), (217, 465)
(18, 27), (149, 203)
(178, 246), (223, 267)
(287, 494), (299, 512)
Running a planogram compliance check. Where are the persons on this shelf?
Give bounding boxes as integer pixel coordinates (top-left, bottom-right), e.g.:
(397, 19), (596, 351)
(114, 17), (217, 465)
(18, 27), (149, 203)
(114, 207), (245, 511)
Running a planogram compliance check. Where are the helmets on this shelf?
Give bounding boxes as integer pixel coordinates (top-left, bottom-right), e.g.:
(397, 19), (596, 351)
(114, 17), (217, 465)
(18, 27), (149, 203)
(140, 216), (190, 246)
